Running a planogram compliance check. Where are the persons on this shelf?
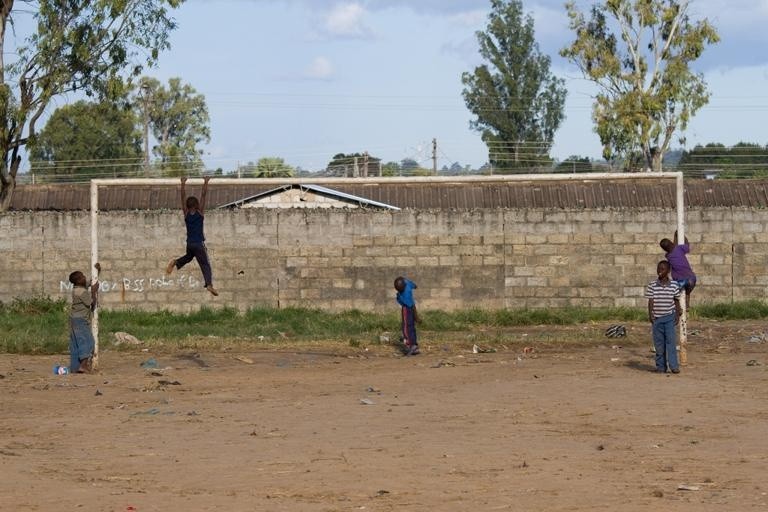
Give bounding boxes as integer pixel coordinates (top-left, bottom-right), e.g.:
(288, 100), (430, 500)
(394, 276), (423, 355)
(68, 262), (102, 374)
(660, 229), (697, 313)
(644, 259), (682, 374)
(165, 176), (220, 297)
